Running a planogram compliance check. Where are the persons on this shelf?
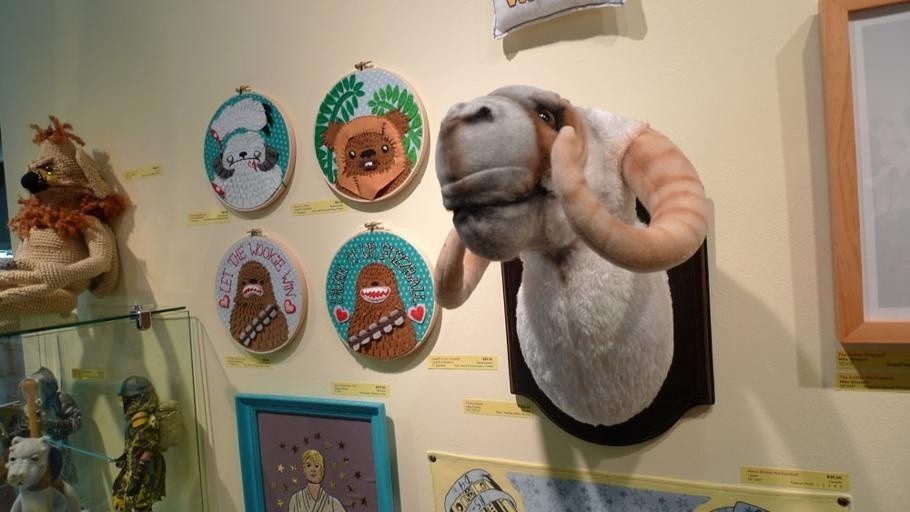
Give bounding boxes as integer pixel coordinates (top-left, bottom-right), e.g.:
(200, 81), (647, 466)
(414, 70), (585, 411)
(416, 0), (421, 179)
(107, 375), (167, 512)
(16, 361), (84, 486)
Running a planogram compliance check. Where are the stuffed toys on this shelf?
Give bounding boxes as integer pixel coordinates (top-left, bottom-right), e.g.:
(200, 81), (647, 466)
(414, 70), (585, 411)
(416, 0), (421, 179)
(0, 112), (132, 320)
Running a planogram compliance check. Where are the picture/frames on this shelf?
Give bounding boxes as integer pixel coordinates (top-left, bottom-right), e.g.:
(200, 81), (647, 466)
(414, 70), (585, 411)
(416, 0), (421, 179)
(816, 0), (910, 346)
(234, 393), (396, 512)
